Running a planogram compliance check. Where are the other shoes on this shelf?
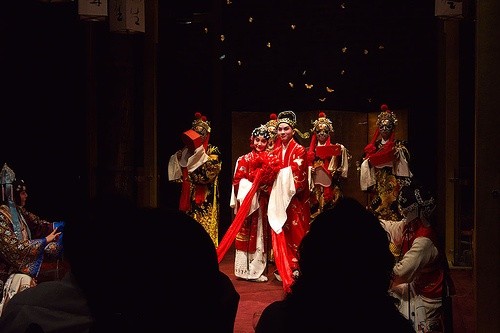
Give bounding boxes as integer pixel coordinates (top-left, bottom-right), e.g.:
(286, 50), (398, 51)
(273, 268), (300, 282)
(250, 274), (268, 282)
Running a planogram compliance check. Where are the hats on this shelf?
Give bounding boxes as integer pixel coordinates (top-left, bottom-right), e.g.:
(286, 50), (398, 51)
(275, 110), (297, 128)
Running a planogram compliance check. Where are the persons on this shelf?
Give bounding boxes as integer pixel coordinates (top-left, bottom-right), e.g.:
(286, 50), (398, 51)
(0, 158), (240, 333)
(168, 112), (222, 250)
(216, 102), (458, 333)
